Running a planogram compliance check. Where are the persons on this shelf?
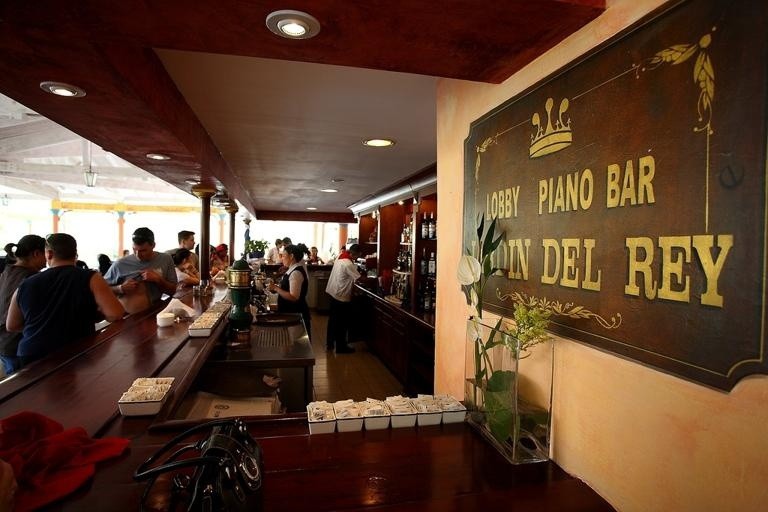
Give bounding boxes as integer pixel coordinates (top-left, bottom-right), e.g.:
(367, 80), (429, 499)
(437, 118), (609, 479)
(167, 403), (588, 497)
(76, 232), (346, 290)
(325, 244), (371, 353)
(265, 243), (310, 345)
(103, 228), (178, 318)
(0, 235), (46, 375)
(6, 233), (125, 369)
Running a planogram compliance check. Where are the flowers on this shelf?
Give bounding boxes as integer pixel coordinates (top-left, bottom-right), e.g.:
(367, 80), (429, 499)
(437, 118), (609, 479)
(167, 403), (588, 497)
(452, 211), (556, 463)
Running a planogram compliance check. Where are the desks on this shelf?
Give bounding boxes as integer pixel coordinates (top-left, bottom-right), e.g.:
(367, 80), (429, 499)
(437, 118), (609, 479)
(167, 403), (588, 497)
(208, 316), (316, 411)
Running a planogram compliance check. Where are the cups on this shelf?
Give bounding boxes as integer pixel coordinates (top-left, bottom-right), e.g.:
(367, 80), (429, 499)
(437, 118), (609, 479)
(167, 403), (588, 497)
(157, 313), (174, 327)
(216, 278), (224, 284)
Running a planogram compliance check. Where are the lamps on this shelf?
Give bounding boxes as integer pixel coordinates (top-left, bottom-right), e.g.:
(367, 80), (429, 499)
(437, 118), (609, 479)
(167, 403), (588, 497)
(83, 138), (99, 188)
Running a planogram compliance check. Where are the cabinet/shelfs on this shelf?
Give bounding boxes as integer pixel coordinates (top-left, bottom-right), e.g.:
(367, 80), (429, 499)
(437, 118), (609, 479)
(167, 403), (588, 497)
(354, 189), (437, 384)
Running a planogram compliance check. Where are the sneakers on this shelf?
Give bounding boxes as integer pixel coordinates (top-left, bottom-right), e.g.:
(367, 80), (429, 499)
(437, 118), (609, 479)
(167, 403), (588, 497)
(335, 346), (356, 354)
(326, 343), (335, 350)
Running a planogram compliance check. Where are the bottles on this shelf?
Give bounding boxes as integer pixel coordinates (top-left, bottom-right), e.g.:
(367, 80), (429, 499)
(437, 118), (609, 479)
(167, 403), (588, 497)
(228, 260), (254, 345)
(391, 210), (435, 314)
(193, 281), (214, 297)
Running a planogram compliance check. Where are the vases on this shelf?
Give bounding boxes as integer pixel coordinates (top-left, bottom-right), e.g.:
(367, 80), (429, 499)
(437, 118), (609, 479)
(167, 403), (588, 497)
(433, 316), (566, 465)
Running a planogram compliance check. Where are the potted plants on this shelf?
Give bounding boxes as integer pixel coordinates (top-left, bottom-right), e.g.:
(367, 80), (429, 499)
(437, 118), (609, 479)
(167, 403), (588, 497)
(241, 238), (271, 259)
